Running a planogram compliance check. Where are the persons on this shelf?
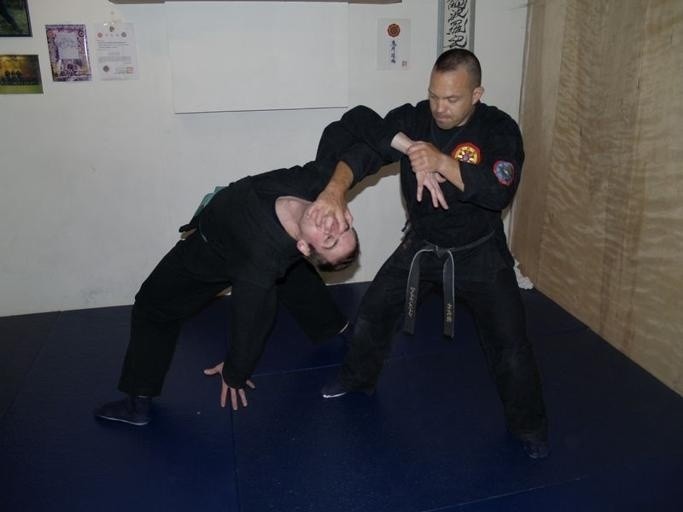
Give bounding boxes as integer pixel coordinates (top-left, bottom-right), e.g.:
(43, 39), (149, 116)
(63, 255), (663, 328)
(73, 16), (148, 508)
(97, 104), (448, 425)
(308, 48), (549, 459)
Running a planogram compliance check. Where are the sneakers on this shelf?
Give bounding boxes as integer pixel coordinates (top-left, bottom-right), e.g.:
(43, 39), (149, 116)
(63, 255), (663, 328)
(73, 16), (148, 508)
(527, 437), (550, 459)
(320, 380), (374, 399)
(96, 397), (152, 426)
